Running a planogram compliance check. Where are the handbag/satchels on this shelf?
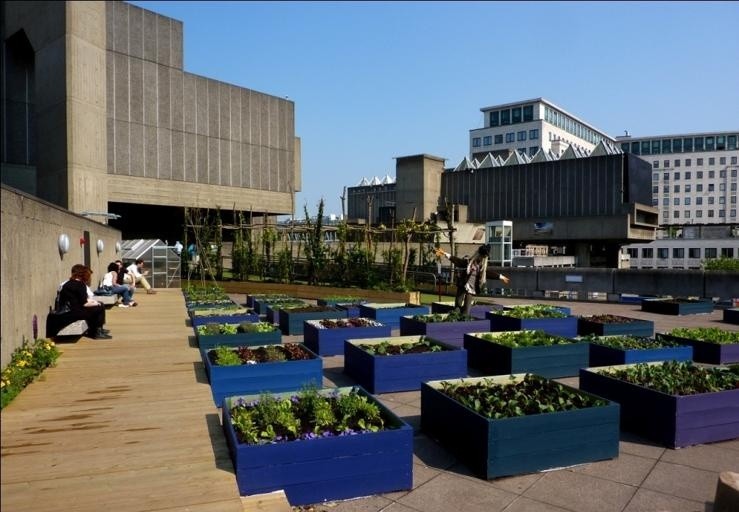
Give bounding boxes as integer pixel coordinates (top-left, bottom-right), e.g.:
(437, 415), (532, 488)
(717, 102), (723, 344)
(93, 278), (109, 295)
(47, 302), (71, 337)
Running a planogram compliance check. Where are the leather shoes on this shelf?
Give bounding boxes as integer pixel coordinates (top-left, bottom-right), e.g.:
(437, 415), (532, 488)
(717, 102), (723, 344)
(94, 326), (112, 339)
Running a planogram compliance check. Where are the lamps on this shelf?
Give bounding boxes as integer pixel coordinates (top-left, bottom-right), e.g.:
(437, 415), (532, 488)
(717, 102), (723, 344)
(115, 241), (122, 253)
(57, 233), (71, 255)
(96, 239), (104, 252)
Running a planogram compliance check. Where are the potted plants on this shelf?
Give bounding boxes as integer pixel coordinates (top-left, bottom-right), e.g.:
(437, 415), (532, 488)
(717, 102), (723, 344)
(639, 297), (715, 315)
(568, 334), (694, 368)
(580, 314), (656, 338)
(431, 300), (571, 320)
(489, 310), (581, 340)
(193, 324), (283, 362)
(217, 383), (415, 507)
(184, 287), (260, 345)
(318, 295), (431, 330)
(202, 342), (325, 408)
(421, 373), (623, 483)
(305, 317), (391, 356)
(246, 291), (349, 336)
(463, 329), (588, 379)
(342, 335), (469, 394)
(400, 314), (491, 344)
(654, 328), (738, 364)
(577, 358), (737, 450)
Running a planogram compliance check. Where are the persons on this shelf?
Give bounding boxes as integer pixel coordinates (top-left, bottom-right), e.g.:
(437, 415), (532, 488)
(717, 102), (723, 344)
(431, 244), (509, 318)
(163, 236), (213, 271)
(50, 265), (113, 340)
(56, 263), (112, 334)
(113, 259), (137, 306)
(126, 257), (158, 295)
(100, 262), (135, 308)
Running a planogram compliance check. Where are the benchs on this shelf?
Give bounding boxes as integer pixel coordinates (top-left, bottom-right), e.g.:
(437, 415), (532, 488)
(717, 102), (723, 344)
(92, 291), (119, 306)
(45, 307), (90, 339)
